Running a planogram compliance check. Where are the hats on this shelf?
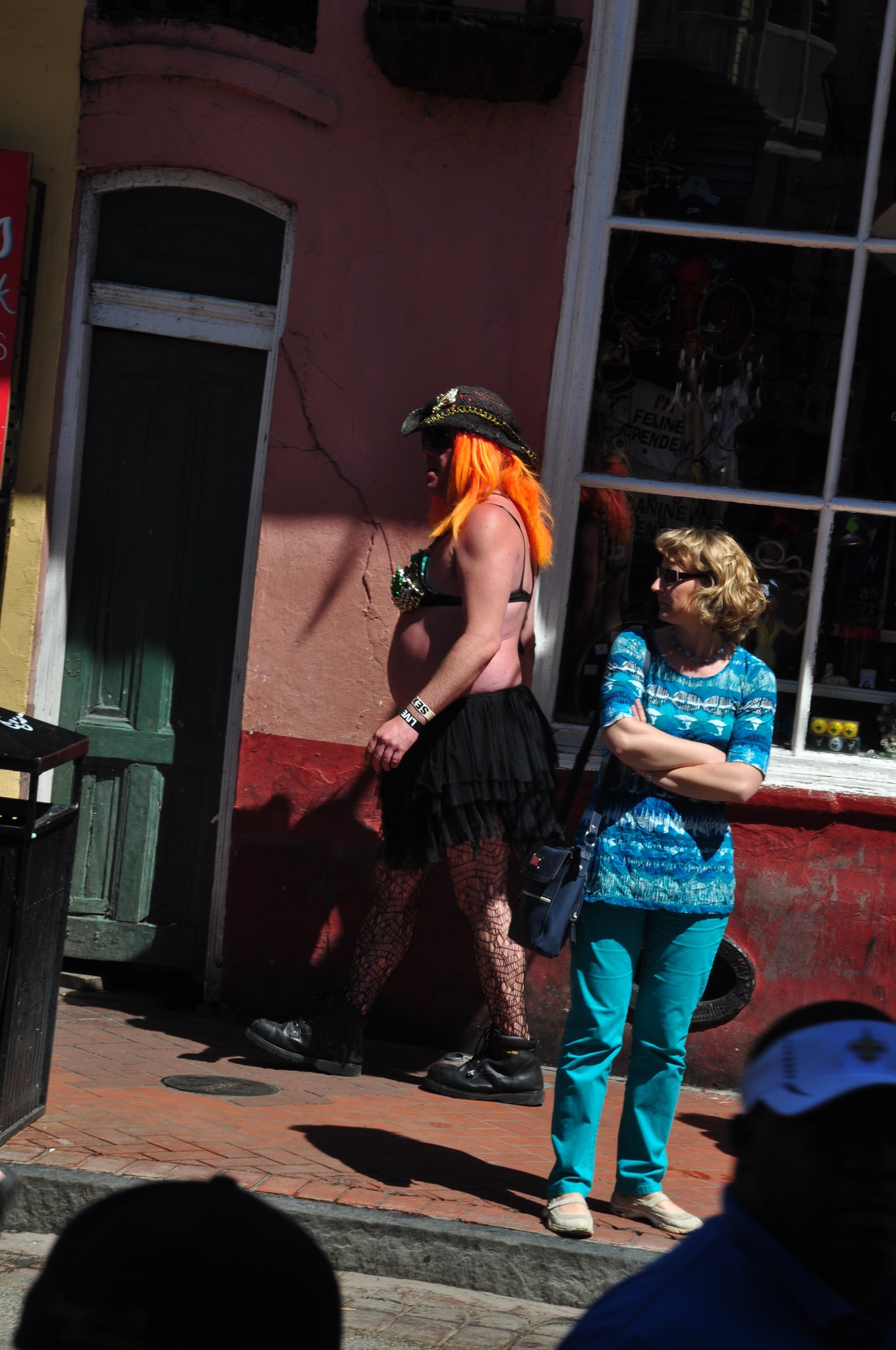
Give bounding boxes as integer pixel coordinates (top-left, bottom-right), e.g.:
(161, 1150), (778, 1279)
(739, 1019), (895, 1118)
(401, 386), (542, 472)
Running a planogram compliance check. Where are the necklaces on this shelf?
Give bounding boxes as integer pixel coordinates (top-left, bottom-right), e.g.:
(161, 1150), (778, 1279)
(669, 625), (729, 665)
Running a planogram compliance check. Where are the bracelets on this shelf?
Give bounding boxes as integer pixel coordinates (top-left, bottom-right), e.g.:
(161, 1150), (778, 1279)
(412, 700), (436, 723)
(644, 770), (656, 783)
(400, 707), (424, 734)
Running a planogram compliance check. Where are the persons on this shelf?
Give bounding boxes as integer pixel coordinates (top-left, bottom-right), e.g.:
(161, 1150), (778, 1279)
(542, 531), (780, 1242)
(8, 1172), (350, 1350)
(557, 993), (893, 1350)
(243, 382), (566, 1106)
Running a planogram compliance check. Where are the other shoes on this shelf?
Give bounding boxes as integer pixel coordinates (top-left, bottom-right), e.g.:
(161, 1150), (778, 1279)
(426, 1023), (545, 1106)
(244, 1003), (364, 1076)
(542, 1193), (595, 1237)
(610, 1185), (704, 1234)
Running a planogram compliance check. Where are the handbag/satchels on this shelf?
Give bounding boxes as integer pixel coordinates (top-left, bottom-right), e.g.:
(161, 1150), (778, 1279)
(508, 832), (595, 959)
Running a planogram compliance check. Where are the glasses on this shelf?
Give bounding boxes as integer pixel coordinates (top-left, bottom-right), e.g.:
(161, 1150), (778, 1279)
(421, 429), (453, 453)
(656, 563), (714, 583)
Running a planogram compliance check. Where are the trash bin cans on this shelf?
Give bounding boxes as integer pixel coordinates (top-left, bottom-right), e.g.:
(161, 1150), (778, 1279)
(0, 704), (89, 1151)
